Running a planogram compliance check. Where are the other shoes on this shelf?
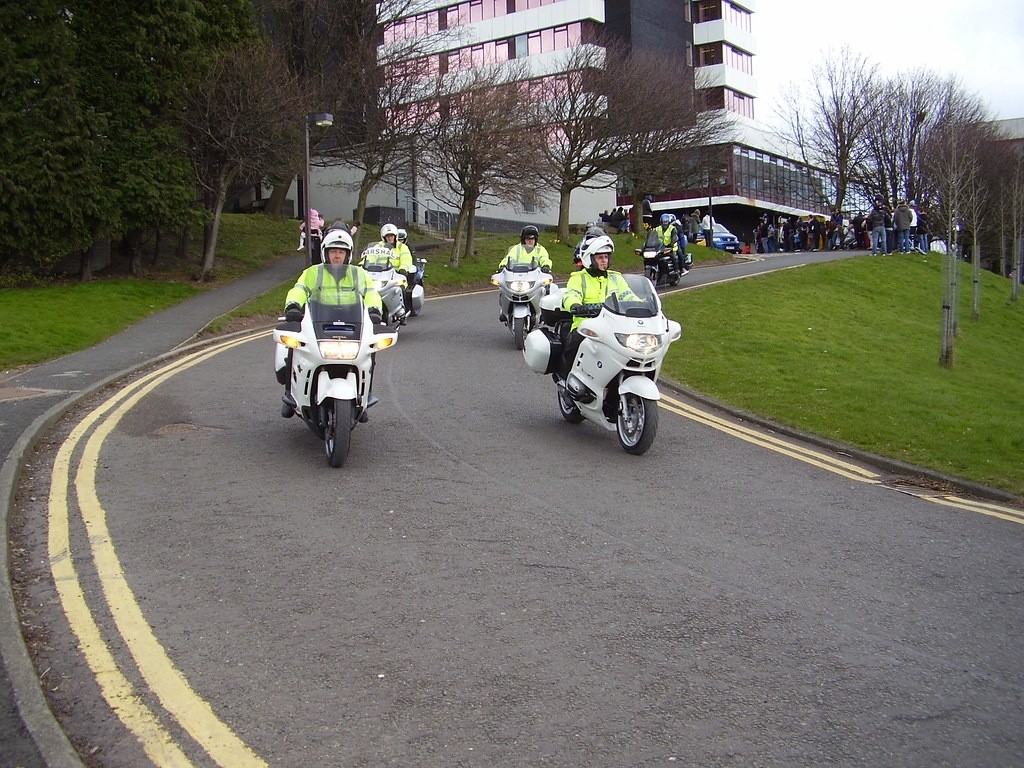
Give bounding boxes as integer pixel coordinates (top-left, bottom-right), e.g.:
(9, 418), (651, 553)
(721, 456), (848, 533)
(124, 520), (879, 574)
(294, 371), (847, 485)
(887, 253), (893, 256)
(812, 249), (817, 252)
(898, 251), (903, 254)
(869, 253), (876, 256)
(868, 247), (873, 250)
(817, 249), (819, 252)
(297, 246), (304, 251)
(906, 251), (910, 254)
(881, 253), (886, 256)
(795, 249), (801, 253)
(779, 249), (785, 252)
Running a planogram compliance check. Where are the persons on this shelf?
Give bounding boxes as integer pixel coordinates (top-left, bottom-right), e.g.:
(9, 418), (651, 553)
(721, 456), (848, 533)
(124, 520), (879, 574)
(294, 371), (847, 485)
(498, 226), (552, 323)
(562, 227), (643, 376)
(753, 197), (928, 256)
(359, 224), (412, 326)
(281, 221), (383, 424)
(702, 212), (715, 247)
(656, 213), (690, 274)
(598, 206), (634, 232)
(641, 195), (652, 215)
(690, 209), (701, 244)
(397, 229), (417, 266)
(297, 209), (324, 250)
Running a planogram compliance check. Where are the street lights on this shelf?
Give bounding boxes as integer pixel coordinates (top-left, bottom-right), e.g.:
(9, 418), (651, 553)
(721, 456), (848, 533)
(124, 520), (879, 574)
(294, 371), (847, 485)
(304, 114), (335, 268)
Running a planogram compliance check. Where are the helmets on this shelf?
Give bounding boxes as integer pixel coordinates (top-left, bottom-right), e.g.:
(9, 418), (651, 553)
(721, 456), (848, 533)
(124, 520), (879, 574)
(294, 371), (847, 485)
(580, 235), (614, 275)
(669, 214), (676, 223)
(521, 225), (538, 244)
(381, 224), (398, 242)
(645, 195), (652, 201)
(321, 230), (354, 264)
(660, 214), (671, 228)
(586, 222), (594, 230)
(398, 229), (408, 244)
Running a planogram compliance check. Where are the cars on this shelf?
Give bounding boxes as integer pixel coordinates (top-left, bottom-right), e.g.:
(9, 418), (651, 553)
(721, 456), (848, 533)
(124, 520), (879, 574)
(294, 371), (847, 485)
(697, 221), (740, 254)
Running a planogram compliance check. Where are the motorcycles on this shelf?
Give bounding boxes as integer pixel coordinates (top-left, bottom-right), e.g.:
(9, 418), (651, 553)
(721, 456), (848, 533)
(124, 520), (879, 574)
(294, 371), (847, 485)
(523, 273), (683, 455)
(633, 230), (693, 288)
(491, 245), (553, 350)
(357, 241), (427, 332)
(272, 264), (398, 467)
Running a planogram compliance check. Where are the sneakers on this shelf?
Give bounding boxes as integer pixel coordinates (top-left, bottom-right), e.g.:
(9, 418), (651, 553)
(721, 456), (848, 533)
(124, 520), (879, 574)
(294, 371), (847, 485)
(357, 411), (369, 423)
(282, 404), (294, 418)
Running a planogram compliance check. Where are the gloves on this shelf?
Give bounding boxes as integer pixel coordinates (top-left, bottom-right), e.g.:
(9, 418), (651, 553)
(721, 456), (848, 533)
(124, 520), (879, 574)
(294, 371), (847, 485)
(667, 243), (674, 247)
(543, 266), (549, 273)
(399, 270), (408, 277)
(499, 267), (503, 272)
(370, 307), (381, 315)
(285, 306), (303, 322)
(570, 304), (590, 317)
